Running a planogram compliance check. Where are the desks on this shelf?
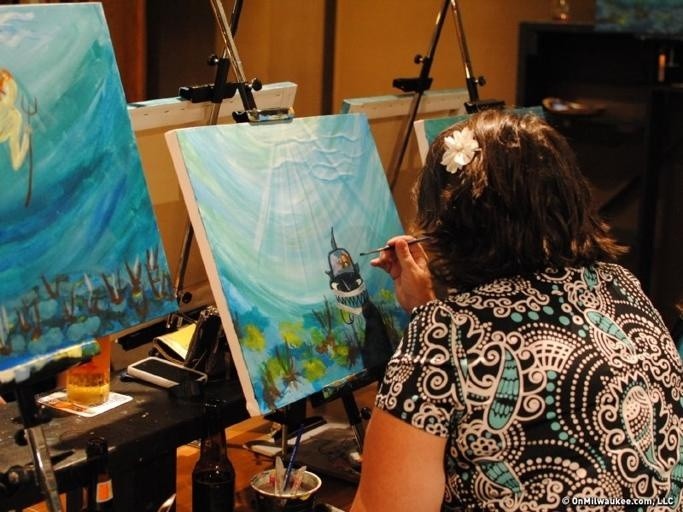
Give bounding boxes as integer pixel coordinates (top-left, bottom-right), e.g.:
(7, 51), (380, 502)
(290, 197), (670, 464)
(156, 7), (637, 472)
(1, 310), (247, 511)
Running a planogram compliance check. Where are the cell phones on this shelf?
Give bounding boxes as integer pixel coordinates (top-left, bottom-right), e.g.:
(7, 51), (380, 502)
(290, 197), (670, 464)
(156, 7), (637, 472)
(127, 357), (206, 390)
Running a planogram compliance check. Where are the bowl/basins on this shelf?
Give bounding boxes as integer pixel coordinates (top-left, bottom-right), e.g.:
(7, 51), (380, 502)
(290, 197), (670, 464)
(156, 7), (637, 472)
(251, 469), (323, 511)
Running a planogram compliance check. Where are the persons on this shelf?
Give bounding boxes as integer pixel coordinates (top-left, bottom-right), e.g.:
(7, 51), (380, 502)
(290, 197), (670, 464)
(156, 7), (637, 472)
(349, 111), (683, 512)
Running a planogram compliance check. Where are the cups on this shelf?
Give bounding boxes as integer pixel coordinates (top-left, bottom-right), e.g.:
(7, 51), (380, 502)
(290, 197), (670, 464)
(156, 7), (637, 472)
(68, 335), (114, 407)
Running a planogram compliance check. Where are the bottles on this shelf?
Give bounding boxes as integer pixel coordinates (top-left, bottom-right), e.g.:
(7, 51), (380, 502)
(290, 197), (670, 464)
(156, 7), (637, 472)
(191, 398), (235, 512)
(79, 438), (114, 512)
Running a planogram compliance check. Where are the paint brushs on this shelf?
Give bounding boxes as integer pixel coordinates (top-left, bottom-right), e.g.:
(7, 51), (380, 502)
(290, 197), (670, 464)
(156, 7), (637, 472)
(281, 423), (303, 493)
(361, 233), (432, 257)
